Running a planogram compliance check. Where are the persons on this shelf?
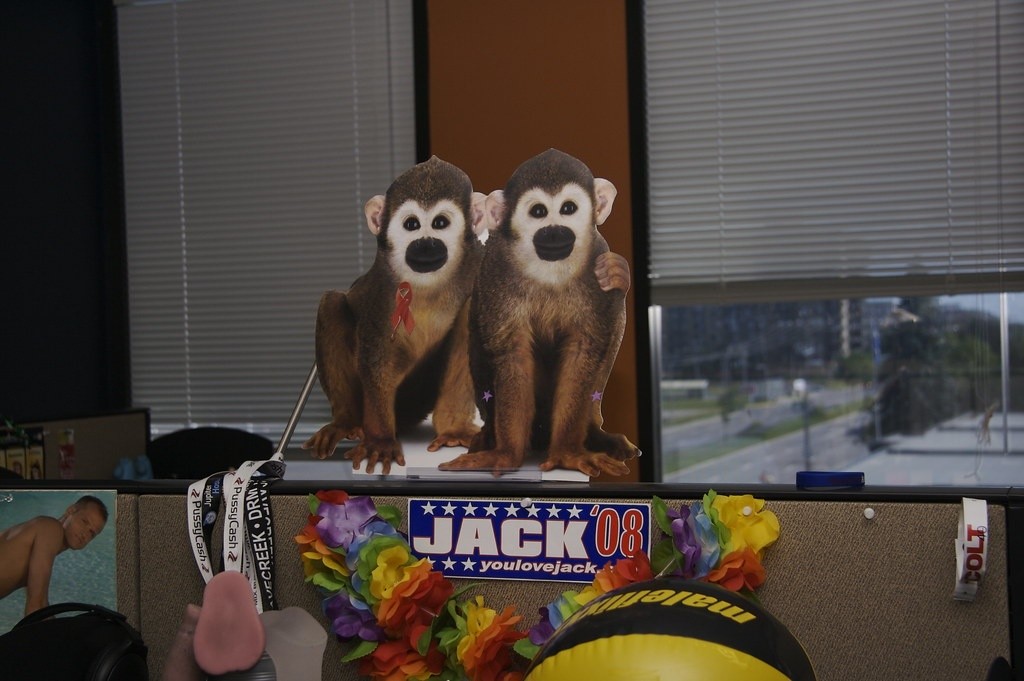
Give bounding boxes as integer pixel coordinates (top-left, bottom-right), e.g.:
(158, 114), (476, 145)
(0, 495), (110, 617)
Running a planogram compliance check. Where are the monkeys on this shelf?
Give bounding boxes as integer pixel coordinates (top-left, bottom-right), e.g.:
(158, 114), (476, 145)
(435, 148), (643, 479)
(302, 155), (631, 468)
